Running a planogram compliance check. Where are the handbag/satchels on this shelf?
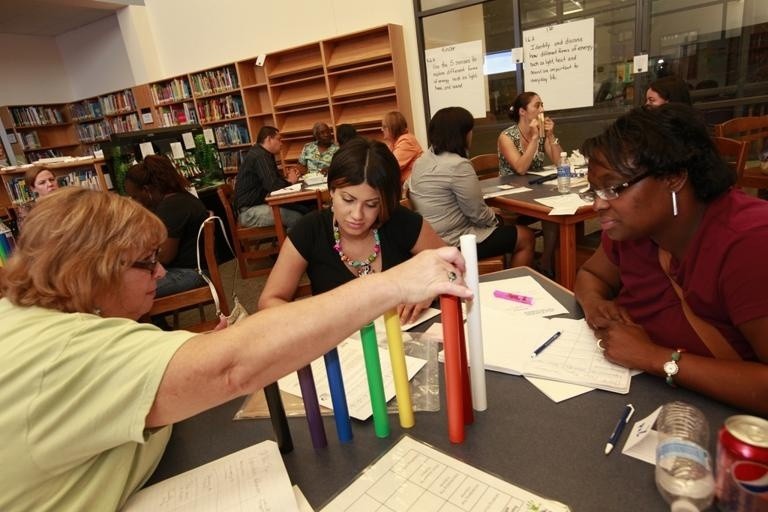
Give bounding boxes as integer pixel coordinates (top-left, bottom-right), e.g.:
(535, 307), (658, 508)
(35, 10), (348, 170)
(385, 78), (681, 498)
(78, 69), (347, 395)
(217, 296), (248, 326)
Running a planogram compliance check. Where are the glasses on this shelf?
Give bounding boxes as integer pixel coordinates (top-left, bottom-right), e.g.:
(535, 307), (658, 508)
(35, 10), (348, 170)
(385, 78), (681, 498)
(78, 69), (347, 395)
(117, 246), (163, 272)
(579, 169), (651, 203)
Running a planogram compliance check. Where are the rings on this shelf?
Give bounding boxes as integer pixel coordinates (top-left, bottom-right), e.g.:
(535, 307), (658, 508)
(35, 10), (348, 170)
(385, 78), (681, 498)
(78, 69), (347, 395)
(596, 338), (605, 354)
(448, 272), (457, 282)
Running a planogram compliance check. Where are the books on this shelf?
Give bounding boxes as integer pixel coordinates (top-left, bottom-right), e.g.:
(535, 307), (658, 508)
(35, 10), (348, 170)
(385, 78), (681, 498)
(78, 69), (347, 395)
(439, 304), (631, 395)
(151, 68), (248, 189)
(1, 105), (95, 170)
(56, 170), (102, 190)
(0, 178), (38, 267)
(70, 90), (140, 158)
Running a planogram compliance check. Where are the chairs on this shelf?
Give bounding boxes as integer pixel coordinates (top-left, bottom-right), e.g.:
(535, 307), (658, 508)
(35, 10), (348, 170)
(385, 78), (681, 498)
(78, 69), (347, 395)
(280, 164), (307, 178)
(398, 197), (505, 275)
(314, 187), (333, 213)
(471, 154), (503, 181)
(216, 184), (283, 279)
(709, 135), (748, 188)
(715, 116), (768, 189)
(143, 210), (230, 328)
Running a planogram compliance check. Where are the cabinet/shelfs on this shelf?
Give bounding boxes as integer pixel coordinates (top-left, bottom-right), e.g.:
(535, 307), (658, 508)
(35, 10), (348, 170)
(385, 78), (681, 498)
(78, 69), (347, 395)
(100, 122), (238, 269)
(0, 104), (90, 167)
(0, 156), (109, 245)
(60, 22), (414, 213)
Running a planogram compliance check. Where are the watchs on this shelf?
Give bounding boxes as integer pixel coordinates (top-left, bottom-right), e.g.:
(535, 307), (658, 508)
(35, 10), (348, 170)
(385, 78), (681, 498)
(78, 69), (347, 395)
(663, 347), (687, 389)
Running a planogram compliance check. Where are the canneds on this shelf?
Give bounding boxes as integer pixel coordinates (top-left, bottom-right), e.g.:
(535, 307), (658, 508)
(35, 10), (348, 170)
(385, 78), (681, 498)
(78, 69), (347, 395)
(712, 414), (768, 512)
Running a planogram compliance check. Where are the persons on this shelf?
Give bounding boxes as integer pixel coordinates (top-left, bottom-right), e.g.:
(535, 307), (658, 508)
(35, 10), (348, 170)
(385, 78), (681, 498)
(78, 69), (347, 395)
(122, 152), (208, 337)
(232, 126), (309, 233)
(296, 120), (338, 177)
(381, 111), (424, 183)
(408, 106), (536, 267)
(24, 166), (59, 200)
(337, 124), (357, 145)
(496, 91), (585, 275)
(573, 101), (768, 416)
(257, 135), (449, 318)
(0, 184), (474, 512)
(645, 76), (691, 110)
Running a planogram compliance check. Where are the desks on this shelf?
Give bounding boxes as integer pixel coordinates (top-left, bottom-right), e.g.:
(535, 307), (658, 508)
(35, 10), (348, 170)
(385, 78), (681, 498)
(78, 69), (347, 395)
(475, 170), (609, 293)
(266, 173), (336, 256)
(136, 265), (767, 511)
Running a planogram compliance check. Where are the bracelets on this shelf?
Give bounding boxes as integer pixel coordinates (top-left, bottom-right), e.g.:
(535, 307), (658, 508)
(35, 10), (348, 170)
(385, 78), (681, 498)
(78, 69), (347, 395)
(552, 138), (560, 145)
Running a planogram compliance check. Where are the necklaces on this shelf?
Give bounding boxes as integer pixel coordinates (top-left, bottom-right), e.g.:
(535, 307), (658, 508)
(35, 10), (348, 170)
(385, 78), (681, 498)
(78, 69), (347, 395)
(332, 222), (381, 277)
(518, 126), (530, 144)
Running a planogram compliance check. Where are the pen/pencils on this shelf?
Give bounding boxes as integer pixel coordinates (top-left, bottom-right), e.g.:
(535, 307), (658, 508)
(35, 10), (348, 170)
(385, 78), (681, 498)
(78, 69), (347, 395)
(604, 404), (635, 455)
(530, 330), (563, 359)
(494, 290), (533, 305)
(528, 175), (557, 185)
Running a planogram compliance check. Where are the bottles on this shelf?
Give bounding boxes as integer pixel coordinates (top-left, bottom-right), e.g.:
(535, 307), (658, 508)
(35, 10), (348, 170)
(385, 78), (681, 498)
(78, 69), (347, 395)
(556, 152), (571, 194)
(654, 399), (714, 511)
(759, 140), (768, 176)
